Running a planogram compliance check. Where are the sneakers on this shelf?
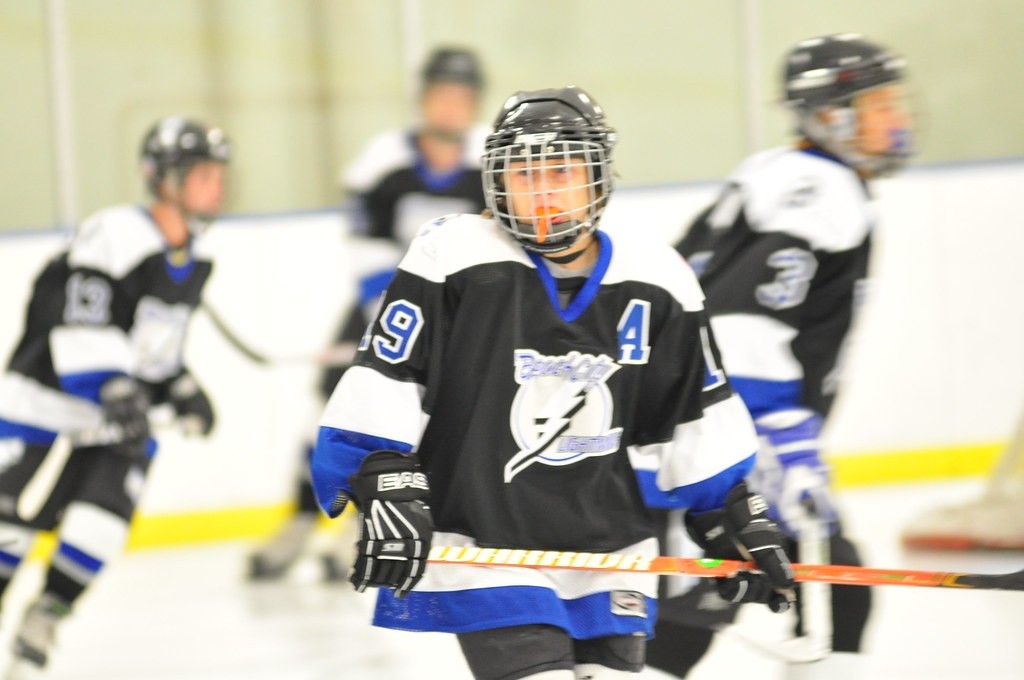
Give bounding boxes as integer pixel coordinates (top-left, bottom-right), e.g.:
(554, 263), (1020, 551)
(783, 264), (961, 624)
(6, 591), (73, 680)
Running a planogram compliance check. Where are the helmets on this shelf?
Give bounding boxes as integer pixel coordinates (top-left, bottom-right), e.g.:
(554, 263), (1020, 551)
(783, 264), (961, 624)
(486, 87), (615, 255)
(779, 33), (914, 176)
(141, 119), (230, 175)
(423, 48), (485, 92)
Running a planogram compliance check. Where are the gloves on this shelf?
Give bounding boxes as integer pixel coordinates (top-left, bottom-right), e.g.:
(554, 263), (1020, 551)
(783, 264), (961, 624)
(97, 376), (150, 455)
(760, 409), (836, 541)
(161, 369), (215, 437)
(350, 449), (435, 600)
(683, 480), (799, 614)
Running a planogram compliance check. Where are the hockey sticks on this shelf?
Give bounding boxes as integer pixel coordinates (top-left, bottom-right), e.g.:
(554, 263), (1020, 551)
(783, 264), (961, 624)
(414, 532), (1024, 600)
(198, 292), (364, 378)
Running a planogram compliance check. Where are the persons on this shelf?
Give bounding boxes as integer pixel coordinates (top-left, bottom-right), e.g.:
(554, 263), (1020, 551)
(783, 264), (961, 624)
(645, 34), (915, 680)
(313, 86), (793, 680)
(246, 48), (484, 580)
(0, 116), (229, 665)
